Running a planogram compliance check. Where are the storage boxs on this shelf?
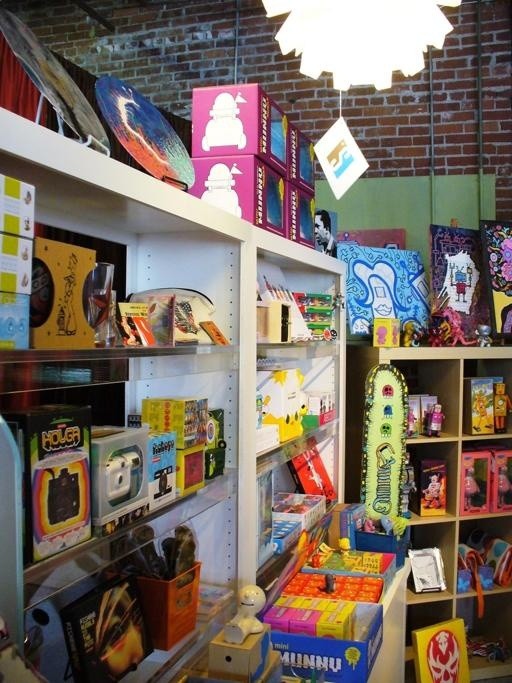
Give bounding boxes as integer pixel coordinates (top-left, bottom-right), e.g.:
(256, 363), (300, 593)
(189, 81), (290, 177)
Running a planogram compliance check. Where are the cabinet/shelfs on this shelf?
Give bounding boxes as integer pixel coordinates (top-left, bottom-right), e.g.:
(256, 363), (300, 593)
(1, 104), (346, 683)
(346, 343), (512, 683)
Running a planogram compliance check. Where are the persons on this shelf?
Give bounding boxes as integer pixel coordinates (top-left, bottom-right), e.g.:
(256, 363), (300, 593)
(316, 210), (337, 256)
